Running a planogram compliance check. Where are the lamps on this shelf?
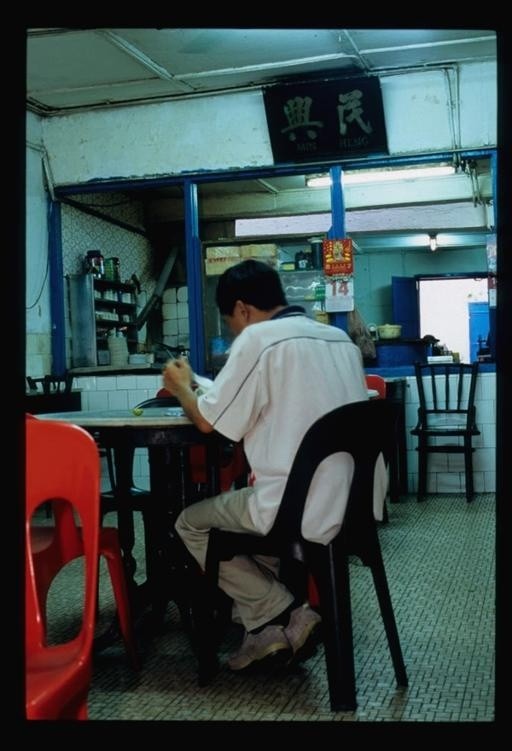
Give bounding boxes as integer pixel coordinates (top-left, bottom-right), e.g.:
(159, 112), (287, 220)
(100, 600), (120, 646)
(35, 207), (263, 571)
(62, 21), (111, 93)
(304, 165), (456, 189)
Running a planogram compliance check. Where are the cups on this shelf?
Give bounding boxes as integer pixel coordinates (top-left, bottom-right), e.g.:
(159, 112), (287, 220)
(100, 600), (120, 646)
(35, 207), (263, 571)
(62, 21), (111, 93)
(94, 288), (133, 322)
(109, 337), (128, 369)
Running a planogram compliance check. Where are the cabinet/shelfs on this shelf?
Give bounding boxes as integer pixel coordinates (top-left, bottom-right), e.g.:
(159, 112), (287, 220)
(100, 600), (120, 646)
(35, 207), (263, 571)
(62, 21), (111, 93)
(68, 273), (139, 369)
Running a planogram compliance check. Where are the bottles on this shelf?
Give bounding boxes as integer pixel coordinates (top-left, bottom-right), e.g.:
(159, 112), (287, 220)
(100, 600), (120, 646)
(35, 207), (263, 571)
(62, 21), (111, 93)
(82, 250), (104, 282)
(370, 326), (377, 341)
(282, 240), (324, 300)
(104, 258), (120, 282)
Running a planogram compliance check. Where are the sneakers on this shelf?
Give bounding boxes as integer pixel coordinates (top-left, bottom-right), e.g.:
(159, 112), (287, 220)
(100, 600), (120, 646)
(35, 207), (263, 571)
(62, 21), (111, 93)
(226, 624), (294, 675)
(273, 606), (326, 674)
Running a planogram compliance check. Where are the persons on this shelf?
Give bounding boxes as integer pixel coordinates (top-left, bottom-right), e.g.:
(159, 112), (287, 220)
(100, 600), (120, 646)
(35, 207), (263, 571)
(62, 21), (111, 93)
(157, 257), (393, 675)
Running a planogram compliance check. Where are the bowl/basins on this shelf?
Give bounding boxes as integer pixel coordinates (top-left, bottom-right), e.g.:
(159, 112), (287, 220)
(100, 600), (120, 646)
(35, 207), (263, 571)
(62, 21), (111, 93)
(379, 324), (402, 338)
(130, 355), (155, 365)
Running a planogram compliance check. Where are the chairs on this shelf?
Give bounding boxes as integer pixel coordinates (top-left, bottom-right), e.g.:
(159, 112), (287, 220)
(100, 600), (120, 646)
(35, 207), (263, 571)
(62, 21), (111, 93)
(365, 373), (387, 400)
(411, 355), (484, 500)
(26, 373), (252, 720)
(197, 397), (409, 712)
